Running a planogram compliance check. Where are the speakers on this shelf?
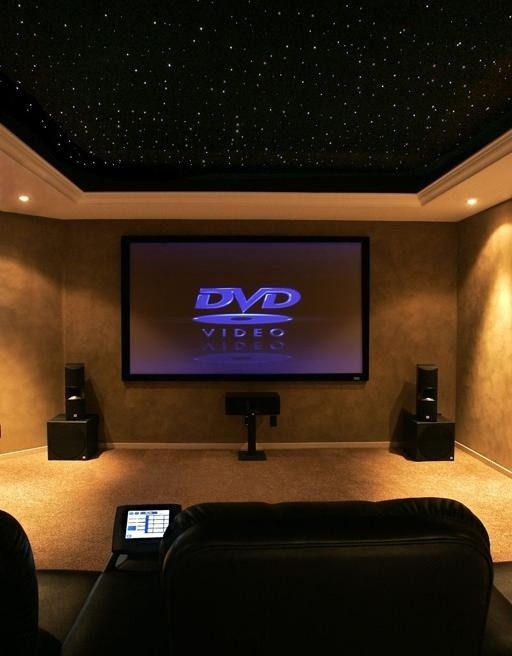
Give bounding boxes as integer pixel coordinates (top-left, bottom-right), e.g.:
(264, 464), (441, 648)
(401, 408), (455, 462)
(415, 364), (438, 422)
(64, 362), (86, 420)
(47, 414), (99, 460)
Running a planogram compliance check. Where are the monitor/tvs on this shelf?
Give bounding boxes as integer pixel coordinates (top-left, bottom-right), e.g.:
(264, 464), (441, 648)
(112, 503), (182, 554)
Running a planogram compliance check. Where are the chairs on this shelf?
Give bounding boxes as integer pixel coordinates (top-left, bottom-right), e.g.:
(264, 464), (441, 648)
(0, 496), (493, 656)
